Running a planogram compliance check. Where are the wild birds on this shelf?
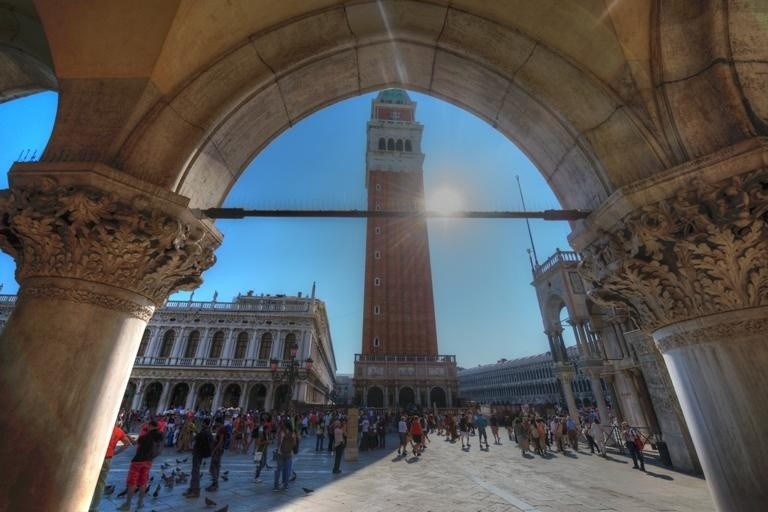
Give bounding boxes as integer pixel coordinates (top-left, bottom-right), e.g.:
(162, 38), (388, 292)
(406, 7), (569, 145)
(104, 483), (117, 496)
(215, 504), (229, 512)
(220, 475), (228, 481)
(199, 471), (204, 479)
(222, 470), (229, 474)
(203, 460), (208, 465)
(204, 497), (217, 506)
(302, 488), (315, 496)
(145, 457), (189, 499)
(119, 490), (127, 497)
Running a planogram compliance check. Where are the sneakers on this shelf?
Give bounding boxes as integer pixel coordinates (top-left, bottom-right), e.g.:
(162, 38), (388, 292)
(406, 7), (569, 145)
(116, 502), (144, 510)
(462, 438), (500, 448)
(182, 486), (218, 498)
(632, 464), (644, 471)
(398, 445), (427, 456)
(252, 472), (296, 492)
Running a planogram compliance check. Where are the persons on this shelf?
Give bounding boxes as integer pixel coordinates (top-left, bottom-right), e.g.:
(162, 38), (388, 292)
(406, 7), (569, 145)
(116, 420), (162, 510)
(620, 421), (645, 471)
(252, 422), (267, 483)
(90, 424), (131, 511)
(204, 415), (232, 491)
(182, 418), (217, 498)
(119, 396), (612, 458)
(271, 419), (295, 490)
(332, 419), (348, 474)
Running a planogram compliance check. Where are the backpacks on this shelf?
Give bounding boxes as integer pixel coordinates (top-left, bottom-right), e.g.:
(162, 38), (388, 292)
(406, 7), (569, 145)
(281, 427), (295, 454)
(224, 424), (233, 449)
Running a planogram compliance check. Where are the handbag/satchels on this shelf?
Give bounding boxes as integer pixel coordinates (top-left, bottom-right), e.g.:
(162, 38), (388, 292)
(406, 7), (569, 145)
(634, 435), (644, 450)
(254, 452), (263, 462)
